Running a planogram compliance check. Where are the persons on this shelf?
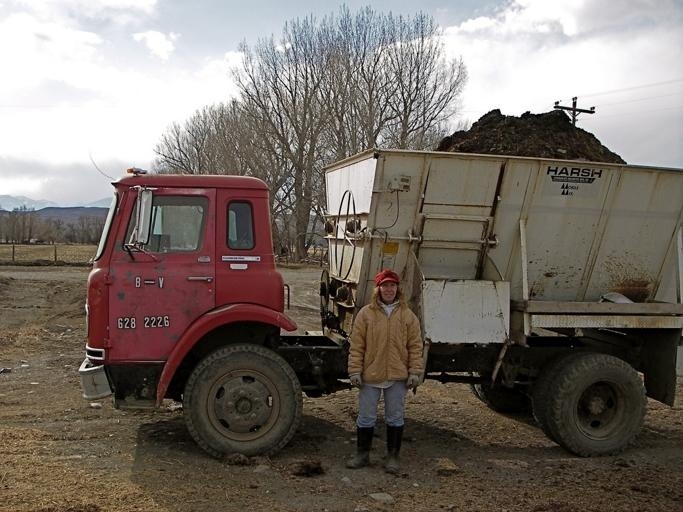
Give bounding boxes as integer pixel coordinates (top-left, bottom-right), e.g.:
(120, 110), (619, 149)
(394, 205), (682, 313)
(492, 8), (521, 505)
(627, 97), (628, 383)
(346, 270), (424, 474)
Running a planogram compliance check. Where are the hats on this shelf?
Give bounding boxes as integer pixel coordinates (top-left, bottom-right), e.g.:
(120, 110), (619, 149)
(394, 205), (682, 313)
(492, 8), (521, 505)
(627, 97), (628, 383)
(375, 270), (400, 286)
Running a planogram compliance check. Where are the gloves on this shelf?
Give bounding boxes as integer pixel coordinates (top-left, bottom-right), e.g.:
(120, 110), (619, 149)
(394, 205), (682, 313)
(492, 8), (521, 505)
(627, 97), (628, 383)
(350, 374), (362, 388)
(406, 374), (419, 389)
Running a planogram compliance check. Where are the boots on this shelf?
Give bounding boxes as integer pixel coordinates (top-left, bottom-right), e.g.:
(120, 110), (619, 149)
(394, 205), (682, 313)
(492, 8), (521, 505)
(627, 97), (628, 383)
(347, 425), (374, 469)
(385, 424), (404, 474)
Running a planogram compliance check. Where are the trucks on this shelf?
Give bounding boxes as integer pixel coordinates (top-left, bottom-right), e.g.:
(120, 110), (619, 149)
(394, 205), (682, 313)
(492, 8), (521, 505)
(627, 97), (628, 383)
(79, 148), (683, 458)
